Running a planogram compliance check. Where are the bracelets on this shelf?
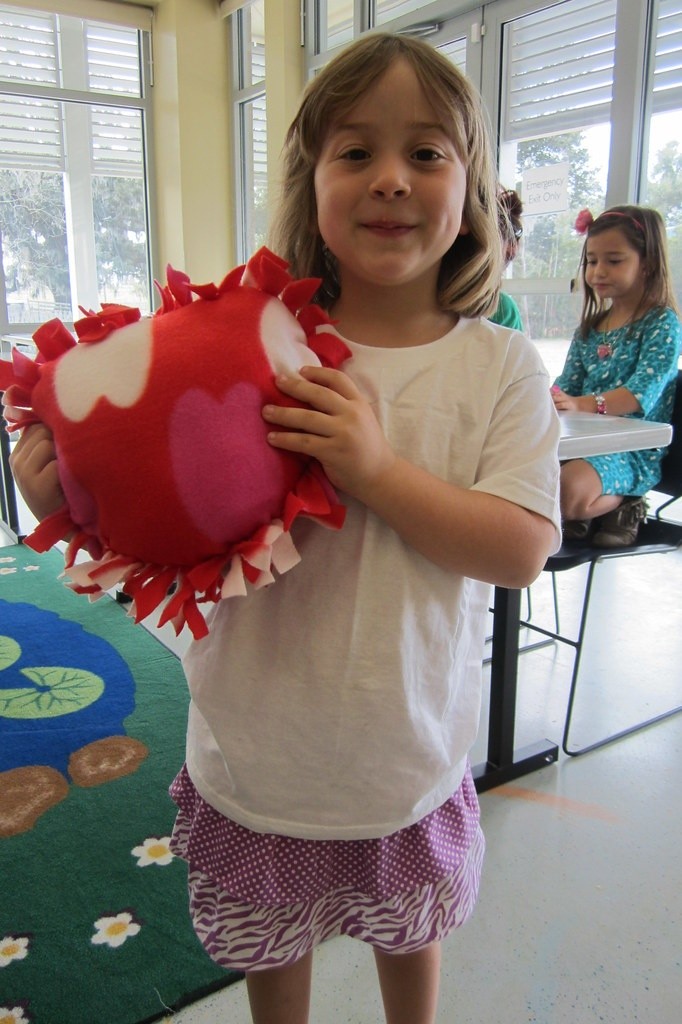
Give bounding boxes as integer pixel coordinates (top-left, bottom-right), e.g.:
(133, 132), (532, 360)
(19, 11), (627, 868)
(593, 392), (607, 414)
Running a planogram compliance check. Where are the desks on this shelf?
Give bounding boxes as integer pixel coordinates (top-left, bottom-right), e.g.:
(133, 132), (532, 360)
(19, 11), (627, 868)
(471, 408), (682, 795)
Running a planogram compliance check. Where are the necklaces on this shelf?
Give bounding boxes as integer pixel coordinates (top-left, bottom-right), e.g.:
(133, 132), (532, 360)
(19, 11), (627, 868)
(598, 306), (633, 359)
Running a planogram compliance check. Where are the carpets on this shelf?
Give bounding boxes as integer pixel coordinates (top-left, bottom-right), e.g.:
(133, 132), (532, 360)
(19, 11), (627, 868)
(0, 545), (245, 1024)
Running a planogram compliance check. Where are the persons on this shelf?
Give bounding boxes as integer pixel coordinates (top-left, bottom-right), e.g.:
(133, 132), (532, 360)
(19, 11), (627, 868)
(8, 35), (562, 1024)
(550, 206), (682, 549)
(487, 190), (524, 332)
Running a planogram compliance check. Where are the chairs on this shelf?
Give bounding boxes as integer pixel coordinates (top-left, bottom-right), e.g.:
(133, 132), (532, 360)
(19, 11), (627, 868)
(487, 367), (682, 756)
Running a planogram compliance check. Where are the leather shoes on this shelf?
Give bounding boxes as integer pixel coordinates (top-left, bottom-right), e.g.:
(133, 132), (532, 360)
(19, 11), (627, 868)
(591, 495), (649, 547)
(561, 517), (594, 541)
